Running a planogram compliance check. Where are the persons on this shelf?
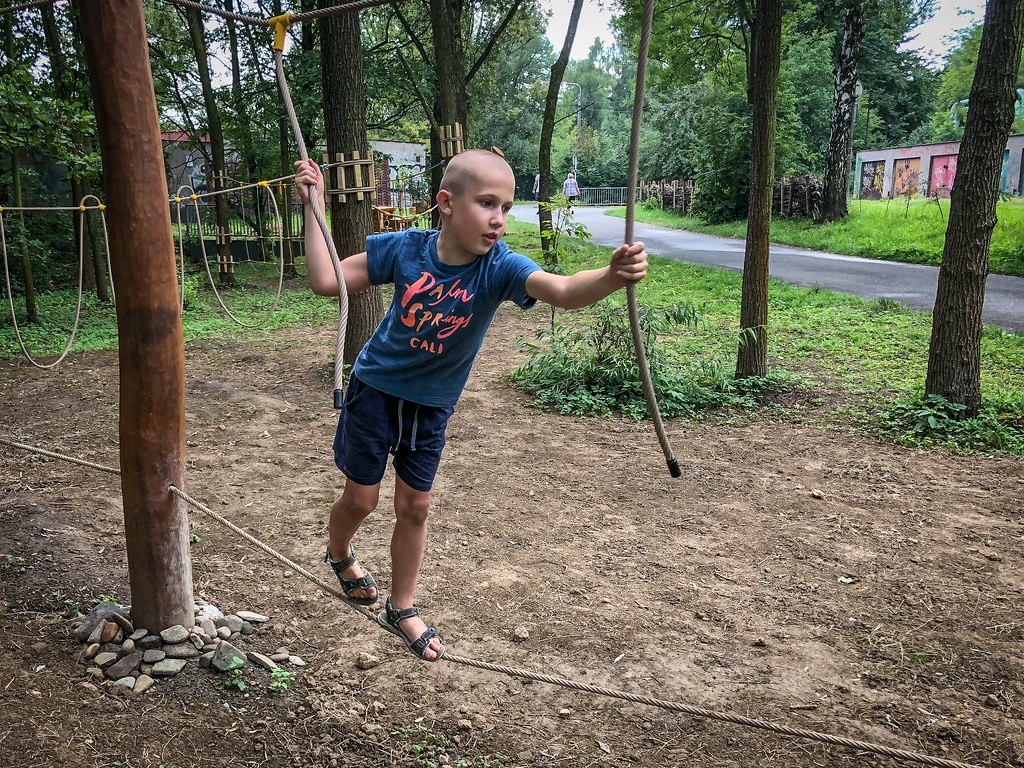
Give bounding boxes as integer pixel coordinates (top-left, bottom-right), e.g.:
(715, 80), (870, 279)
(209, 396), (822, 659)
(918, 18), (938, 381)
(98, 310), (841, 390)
(533, 170), (581, 215)
(293, 148), (648, 661)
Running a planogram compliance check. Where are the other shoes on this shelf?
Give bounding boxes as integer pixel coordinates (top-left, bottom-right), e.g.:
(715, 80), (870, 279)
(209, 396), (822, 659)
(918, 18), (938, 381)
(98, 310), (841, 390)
(536, 211), (539, 214)
(571, 211), (574, 214)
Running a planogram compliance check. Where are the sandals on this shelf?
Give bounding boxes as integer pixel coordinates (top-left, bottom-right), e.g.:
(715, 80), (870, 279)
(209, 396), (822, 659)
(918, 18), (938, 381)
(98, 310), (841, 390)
(377, 593), (446, 662)
(324, 540), (379, 606)
